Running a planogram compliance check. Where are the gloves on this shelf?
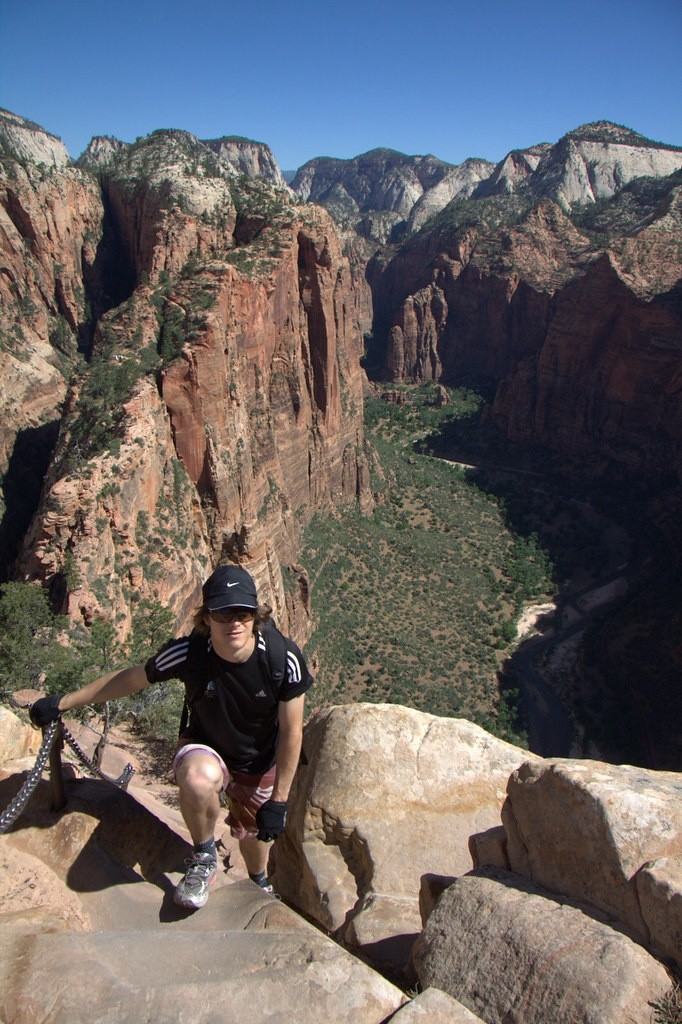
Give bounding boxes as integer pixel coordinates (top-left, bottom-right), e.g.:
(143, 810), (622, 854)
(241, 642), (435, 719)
(256, 799), (287, 842)
(29, 695), (70, 727)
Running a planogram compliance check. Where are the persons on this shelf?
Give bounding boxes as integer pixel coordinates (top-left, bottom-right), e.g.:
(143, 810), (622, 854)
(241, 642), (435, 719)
(28, 564), (316, 908)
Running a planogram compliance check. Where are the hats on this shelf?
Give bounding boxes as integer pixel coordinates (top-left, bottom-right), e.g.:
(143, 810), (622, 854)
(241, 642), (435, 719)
(201, 565), (258, 609)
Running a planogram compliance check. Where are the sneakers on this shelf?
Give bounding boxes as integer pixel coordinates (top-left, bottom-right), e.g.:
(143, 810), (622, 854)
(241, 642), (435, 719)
(263, 884), (281, 901)
(173, 850), (218, 908)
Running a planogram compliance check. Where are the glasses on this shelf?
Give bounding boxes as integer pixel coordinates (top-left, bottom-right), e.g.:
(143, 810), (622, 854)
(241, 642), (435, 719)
(208, 605), (254, 623)
